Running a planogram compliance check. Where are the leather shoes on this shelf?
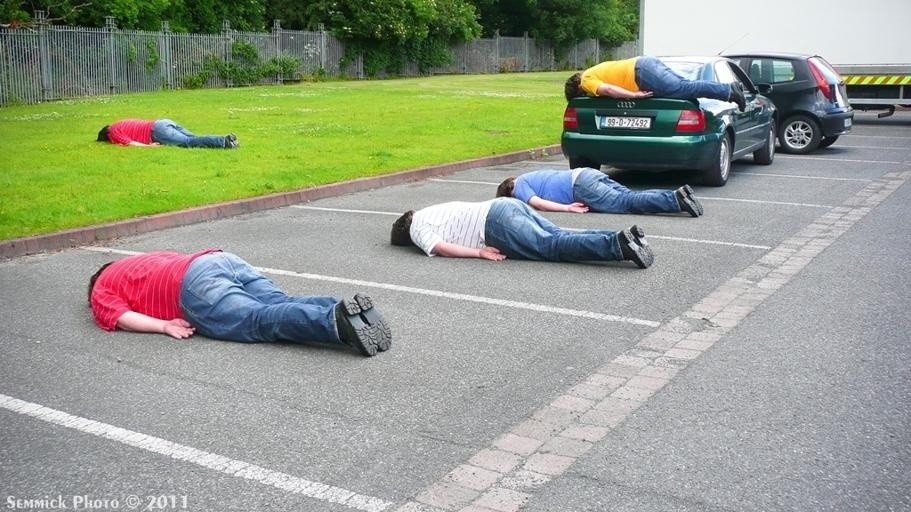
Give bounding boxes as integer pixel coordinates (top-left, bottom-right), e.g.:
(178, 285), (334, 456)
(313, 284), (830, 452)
(337, 296), (377, 356)
(675, 184), (703, 217)
(618, 224), (653, 268)
(225, 133), (240, 148)
(352, 291), (392, 351)
(729, 81), (746, 111)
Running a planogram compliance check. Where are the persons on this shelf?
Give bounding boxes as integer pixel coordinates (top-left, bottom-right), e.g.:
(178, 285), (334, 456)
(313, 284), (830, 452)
(496, 166), (704, 218)
(389, 195), (655, 269)
(94, 118), (240, 151)
(563, 55), (747, 113)
(85, 246), (393, 358)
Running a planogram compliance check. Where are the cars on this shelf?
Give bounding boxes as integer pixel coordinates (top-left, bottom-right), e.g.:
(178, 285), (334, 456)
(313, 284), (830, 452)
(560, 56), (776, 186)
(714, 50), (856, 152)
